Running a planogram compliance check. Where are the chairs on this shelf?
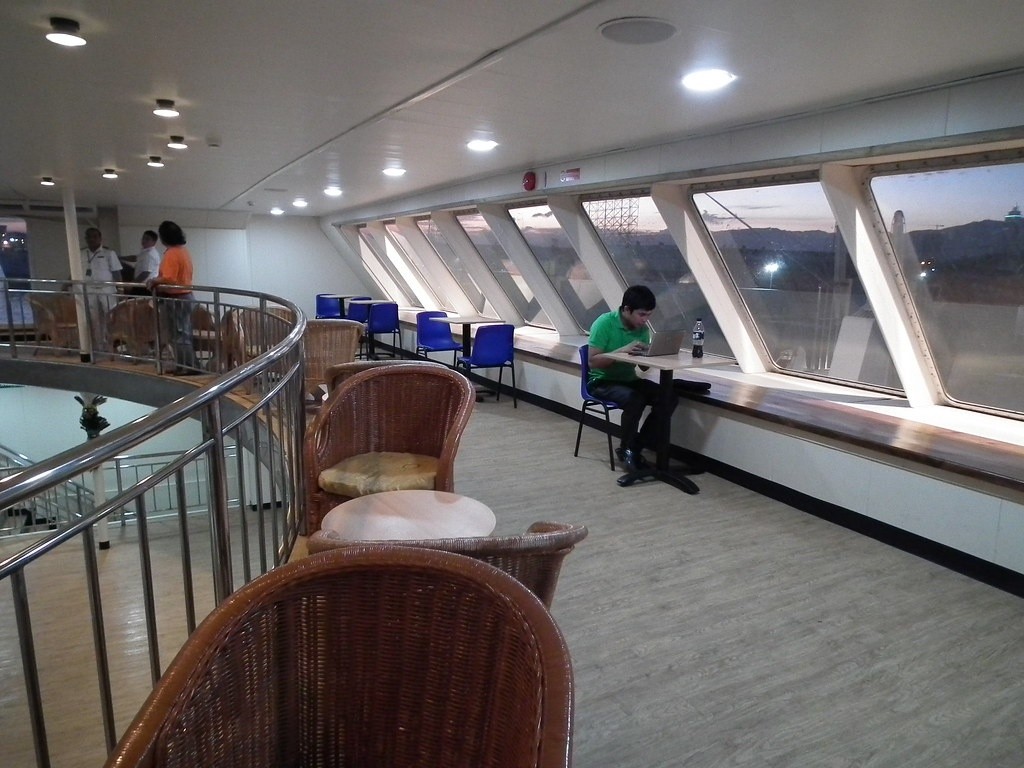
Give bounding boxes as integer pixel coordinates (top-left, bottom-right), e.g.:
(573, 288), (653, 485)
(24, 294), (620, 768)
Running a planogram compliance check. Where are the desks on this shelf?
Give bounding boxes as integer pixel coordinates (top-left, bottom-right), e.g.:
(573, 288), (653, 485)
(321, 489), (496, 538)
(429, 315), (496, 402)
(602, 350), (736, 495)
(321, 295), (355, 319)
(348, 300), (395, 362)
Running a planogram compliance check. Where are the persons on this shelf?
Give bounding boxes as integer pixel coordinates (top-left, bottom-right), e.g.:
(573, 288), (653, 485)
(586, 286), (679, 474)
(79, 228), (123, 352)
(117, 230), (161, 346)
(146, 220), (193, 378)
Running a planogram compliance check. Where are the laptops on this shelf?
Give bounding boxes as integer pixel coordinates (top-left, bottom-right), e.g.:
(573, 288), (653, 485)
(628, 329), (686, 356)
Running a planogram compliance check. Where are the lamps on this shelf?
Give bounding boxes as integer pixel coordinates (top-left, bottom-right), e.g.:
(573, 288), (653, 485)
(40, 177), (54, 186)
(146, 156), (165, 167)
(46, 17), (88, 47)
(152, 99), (179, 118)
(102, 170), (118, 178)
(167, 137), (188, 149)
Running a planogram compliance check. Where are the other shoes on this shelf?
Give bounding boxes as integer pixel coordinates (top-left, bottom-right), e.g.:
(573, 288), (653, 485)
(175, 369), (201, 376)
(616, 445), (648, 468)
(164, 367), (180, 374)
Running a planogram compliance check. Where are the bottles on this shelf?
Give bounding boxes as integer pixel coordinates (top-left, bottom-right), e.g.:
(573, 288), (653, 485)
(691, 317), (705, 358)
